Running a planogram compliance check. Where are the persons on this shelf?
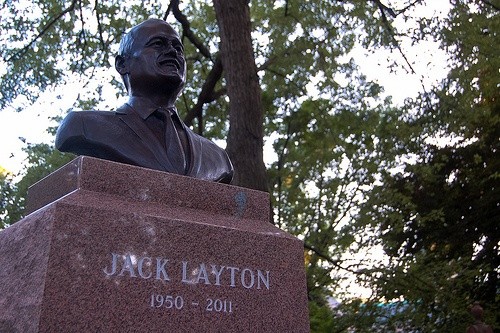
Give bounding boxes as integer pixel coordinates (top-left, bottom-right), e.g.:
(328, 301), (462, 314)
(54, 17), (238, 185)
